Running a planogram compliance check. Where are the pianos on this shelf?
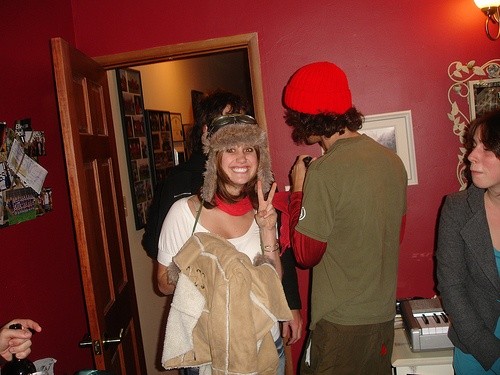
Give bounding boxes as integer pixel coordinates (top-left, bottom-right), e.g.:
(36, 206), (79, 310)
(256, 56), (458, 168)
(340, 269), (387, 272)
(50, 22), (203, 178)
(400, 297), (455, 353)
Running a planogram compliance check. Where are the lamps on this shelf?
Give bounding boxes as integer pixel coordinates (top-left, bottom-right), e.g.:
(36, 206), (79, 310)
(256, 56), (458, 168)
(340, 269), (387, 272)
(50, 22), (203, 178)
(474, 0), (500, 41)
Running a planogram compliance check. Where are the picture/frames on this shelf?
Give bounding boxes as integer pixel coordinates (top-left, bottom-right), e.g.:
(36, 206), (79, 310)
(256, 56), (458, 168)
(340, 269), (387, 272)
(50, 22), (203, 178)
(468, 78), (500, 122)
(356, 110), (419, 185)
(116, 67), (205, 231)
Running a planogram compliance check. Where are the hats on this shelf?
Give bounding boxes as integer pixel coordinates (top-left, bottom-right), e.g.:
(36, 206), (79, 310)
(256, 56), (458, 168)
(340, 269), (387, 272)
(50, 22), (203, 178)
(282, 62), (351, 116)
(201, 114), (274, 202)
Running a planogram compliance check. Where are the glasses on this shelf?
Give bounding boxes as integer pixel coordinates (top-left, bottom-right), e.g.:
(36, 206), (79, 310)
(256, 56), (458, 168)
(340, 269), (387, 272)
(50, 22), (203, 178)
(207, 115), (260, 137)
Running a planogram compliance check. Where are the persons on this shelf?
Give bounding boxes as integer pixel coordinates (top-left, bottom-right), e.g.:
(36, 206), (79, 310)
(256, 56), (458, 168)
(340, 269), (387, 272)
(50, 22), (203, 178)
(156, 115), (287, 375)
(282, 61), (408, 375)
(142, 90), (303, 375)
(436, 109), (500, 375)
(0, 318), (42, 375)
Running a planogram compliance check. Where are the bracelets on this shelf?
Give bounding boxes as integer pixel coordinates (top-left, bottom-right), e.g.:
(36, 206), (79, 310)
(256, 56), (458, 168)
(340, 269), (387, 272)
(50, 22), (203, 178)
(259, 240), (280, 253)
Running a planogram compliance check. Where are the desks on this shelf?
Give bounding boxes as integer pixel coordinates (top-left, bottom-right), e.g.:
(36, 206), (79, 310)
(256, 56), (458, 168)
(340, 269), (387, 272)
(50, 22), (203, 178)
(391, 328), (455, 375)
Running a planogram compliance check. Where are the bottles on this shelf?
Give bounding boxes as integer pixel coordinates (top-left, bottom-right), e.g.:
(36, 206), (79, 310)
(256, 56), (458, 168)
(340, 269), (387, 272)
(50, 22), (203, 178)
(1, 324), (36, 375)
